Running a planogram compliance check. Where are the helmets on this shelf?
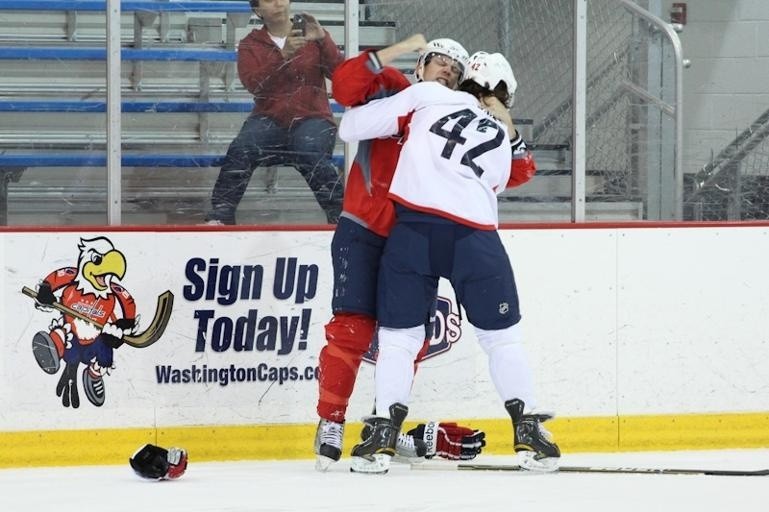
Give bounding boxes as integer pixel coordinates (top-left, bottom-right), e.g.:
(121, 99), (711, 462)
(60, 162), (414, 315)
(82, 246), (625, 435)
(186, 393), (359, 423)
(413, 38), (469, 88)
(458, 50), (518, 113)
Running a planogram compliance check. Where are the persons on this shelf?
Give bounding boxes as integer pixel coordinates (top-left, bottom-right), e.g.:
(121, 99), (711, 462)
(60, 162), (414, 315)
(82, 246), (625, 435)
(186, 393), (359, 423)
(313, 32), (535, 461)
(206, 0), (347, 225)
(337, 51), (563, 461)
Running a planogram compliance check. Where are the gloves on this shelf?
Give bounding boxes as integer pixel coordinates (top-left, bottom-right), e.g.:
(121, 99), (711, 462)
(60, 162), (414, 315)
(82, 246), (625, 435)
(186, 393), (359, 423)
(405, 421), (487, 460)
(129, 444), (190, 481)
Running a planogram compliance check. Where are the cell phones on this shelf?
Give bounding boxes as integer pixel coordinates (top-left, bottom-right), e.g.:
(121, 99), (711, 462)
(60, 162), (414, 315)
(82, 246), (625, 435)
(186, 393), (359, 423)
(293, 14), (305, 36)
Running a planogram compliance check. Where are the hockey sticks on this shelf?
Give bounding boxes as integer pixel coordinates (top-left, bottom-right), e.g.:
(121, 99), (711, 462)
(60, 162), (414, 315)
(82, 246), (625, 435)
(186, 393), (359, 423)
(410, 462), (769, 476)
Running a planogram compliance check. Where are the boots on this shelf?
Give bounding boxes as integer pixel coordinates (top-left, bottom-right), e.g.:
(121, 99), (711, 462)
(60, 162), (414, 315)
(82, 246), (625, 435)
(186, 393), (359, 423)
(312, 414), (346, 473)
(503, 398), (562, 457)
(348, 402), (409, 470)
(360, 402), (428, 459)
